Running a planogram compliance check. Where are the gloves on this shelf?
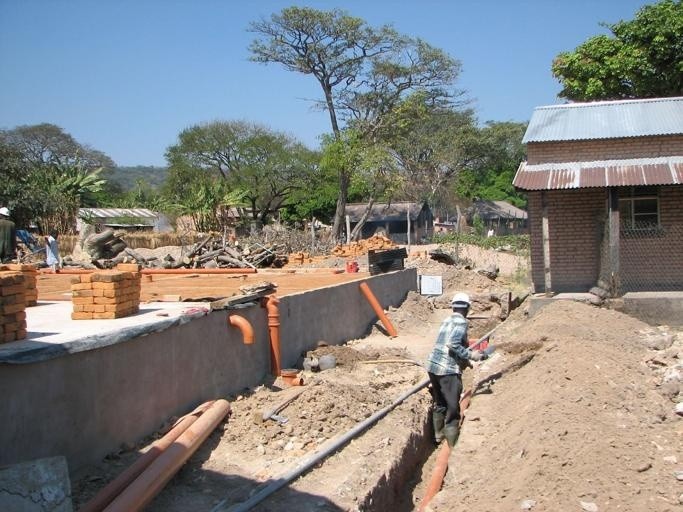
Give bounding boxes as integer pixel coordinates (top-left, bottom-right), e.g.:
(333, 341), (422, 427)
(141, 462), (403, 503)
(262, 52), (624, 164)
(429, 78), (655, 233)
(468, 350), (488, 361)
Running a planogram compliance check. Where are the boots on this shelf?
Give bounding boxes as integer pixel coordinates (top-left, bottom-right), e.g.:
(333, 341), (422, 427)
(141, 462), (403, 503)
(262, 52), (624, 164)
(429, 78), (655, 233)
(445, 426), (460, 447)
(431, 412), (445, 444)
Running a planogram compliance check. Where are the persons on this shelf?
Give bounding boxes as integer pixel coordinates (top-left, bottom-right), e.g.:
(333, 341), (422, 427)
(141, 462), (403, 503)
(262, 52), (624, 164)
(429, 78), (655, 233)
(45, 229), (63, 272)
(425, 294), (488, 447)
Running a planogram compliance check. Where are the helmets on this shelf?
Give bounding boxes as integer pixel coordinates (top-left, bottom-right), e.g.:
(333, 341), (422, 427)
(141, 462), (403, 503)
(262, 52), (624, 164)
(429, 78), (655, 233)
(451, 293), (471, 305)
(0, 207), (10, 216)
